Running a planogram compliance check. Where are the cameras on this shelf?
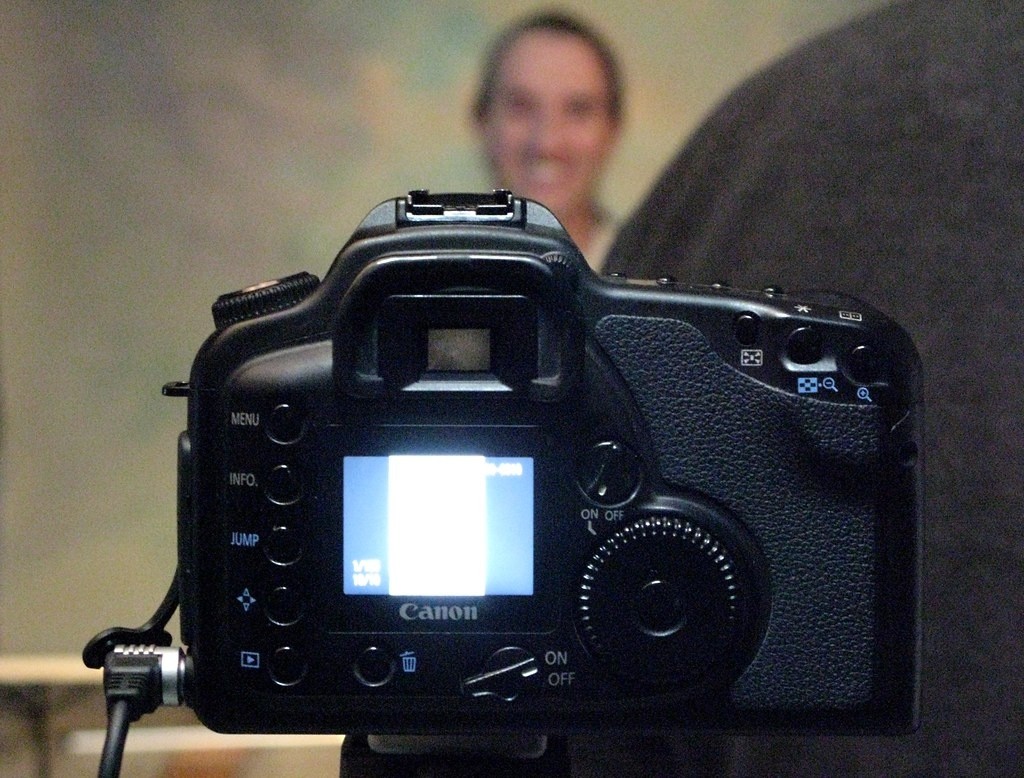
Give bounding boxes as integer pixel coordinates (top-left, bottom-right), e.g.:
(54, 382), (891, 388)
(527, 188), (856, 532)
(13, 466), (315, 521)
(162, 186), (927, 737)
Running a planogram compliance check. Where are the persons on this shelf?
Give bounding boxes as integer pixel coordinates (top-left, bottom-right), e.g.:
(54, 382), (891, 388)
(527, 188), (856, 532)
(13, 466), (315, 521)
(567, 0), (1024, 777)
(470, 9), (624, 276)
